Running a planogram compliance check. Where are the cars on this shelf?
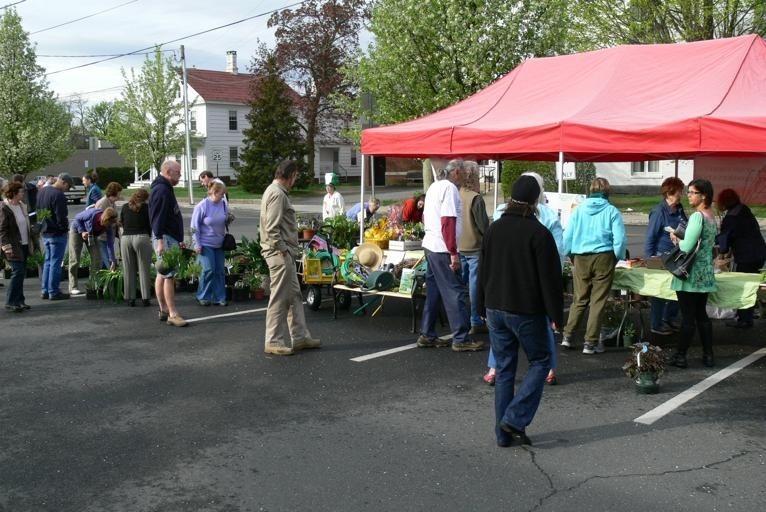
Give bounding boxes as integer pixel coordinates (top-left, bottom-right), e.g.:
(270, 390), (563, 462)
(28, 175), (85, 203)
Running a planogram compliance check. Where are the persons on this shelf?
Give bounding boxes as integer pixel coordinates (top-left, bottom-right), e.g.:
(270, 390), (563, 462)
(254, 161), (322, 356)
(146, 160), (191, 331)
(319, 181), (347, 218)
(418, 158), (482, 351)
(561, 177), (626, 353)
(0, 158), (232, 311)
(642, 177), (766, 367)
(400, 193), (425, 225)
(344, 195), (381, 221)
(482, 170), (563, 385)
(473, 175), (564, 449)
(457, 162), (489, 333)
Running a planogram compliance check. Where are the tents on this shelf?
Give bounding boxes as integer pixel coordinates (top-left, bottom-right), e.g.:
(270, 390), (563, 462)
(360, 34), (766, 246)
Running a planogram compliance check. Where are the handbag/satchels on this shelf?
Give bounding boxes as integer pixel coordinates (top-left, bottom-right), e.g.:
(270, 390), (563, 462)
(223, 234), (237, 251)
(662, 246), (697, 280)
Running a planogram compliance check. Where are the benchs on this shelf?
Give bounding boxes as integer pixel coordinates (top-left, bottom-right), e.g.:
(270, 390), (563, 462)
(332, 249), (425, 333)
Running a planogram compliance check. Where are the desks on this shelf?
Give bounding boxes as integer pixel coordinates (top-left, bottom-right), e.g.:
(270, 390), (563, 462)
(603, 258), (765, 354)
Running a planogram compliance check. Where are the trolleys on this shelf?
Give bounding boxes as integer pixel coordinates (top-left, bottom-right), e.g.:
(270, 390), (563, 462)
(293, 231), (353, 309)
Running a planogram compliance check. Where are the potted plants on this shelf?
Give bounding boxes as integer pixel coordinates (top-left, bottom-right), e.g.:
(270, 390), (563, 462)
(623, 322), (637, 348)
(298, 217), (317, 241)
(1, 208), (270, 300)
(622, 342), (667, 396)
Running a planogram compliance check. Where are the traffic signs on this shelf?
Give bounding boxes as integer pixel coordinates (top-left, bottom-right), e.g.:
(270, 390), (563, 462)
(211, 150), (224, 161)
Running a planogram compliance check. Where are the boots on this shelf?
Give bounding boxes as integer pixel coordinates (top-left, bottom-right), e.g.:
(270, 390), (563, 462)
(663, 326), (696, 367)
(698, 321), (714, 367)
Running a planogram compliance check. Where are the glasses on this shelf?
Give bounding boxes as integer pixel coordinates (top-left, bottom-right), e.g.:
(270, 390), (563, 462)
(685, 191), (700, 195)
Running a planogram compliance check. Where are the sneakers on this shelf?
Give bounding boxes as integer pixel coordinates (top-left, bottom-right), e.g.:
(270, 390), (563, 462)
(500, 421), (532, 446)
(560, 336), (577, 348)
(158, 311), (169, 320)
(416, 335), (449, 348)
(497, 439), (510, 447)
(483, 373), (495, 386)
(546, 374), (557, 385)
(3, 289), (85, 312)
(452, 340), (484, 352)
(264, 346), (294, 355)
(291, 338), (321, 351)
(582, 343), (607, 354)
(196, 299), (211, 306)
(167, 314), (189, 327)
(218, 300), (228, 306)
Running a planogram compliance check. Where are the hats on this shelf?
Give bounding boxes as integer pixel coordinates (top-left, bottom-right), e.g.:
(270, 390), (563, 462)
(354, 243), (382, 272)
(511, 175), (540, 205)
(58, 172), (76, 189)
